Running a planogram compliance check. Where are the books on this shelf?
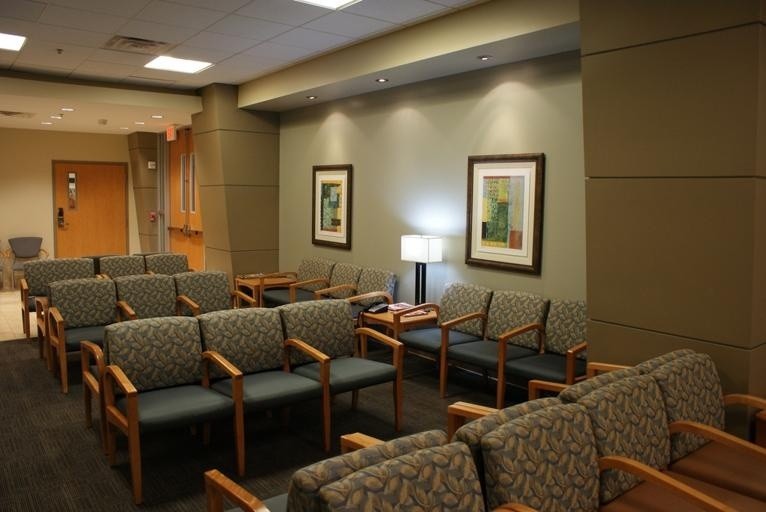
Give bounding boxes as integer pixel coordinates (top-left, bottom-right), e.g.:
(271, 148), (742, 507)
(388, 302), (427, 317)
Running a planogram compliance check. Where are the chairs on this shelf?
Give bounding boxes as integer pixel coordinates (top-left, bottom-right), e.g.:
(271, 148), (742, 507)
(275, 298), (405, 448)
(580, 374), (765, 511)
(438, 290), (551, 407)
(48, 278), (134, 394)
(0, 249), (9, 290)
(145, 254), (195, 274)
(456, 367), (634, 414)
(392, 283), (495, 397)
(101, 317), (246, 506)
(260, 256), (337, 308)
(526, 348), (692, 398)
(174, 271), (257, 314)
(101, 255), (154, 281)
(196, 306), (332, 470)
(2, 235), (52, 291)
(480, 402), (732, 511)
(113, 273), (200, 321)
(21, 258), (101, 340)
(319, 440), (540, 512)
(355, 397), (561, 444)
(289, 263), (364, 305)
(313, 268), (397, 357)
(653, 352), (766, 495)
(496, 301), (588, 408)
(201, 427), (449, 511)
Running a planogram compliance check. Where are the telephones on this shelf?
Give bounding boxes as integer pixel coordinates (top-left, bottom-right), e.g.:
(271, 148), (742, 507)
(357, 301), (388, 328)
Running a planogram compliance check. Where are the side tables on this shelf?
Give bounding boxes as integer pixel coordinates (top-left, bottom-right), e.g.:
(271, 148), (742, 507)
(358, 299), (445, 368)
(236, 271), (294, 311)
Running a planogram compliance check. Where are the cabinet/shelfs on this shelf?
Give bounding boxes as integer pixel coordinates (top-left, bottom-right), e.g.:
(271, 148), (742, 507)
(163, 125), (204, 236)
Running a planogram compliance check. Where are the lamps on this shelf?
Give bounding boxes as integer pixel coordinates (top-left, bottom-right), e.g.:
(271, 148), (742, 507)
(395, 232), (445, 310)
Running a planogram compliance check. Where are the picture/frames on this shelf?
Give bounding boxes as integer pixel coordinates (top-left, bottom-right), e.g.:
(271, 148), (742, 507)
(464, 151), (546, 276)
(309, 163), (356, 252)
